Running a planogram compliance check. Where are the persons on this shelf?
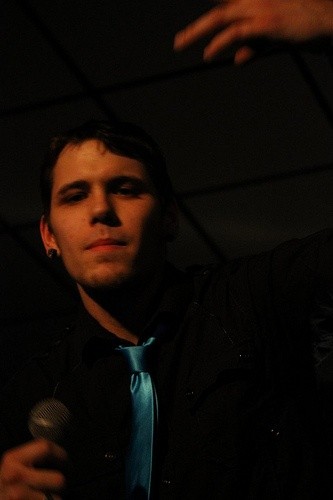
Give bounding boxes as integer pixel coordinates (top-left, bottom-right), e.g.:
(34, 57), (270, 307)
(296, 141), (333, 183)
(1, 0), (333, 500)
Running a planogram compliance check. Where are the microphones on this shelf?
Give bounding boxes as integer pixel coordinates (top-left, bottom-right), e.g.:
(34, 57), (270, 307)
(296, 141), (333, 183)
(29, 399), (72, 500)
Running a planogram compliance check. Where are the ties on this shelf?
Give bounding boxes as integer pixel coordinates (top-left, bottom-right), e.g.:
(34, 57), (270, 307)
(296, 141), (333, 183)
(110, 337), (159, 500)
